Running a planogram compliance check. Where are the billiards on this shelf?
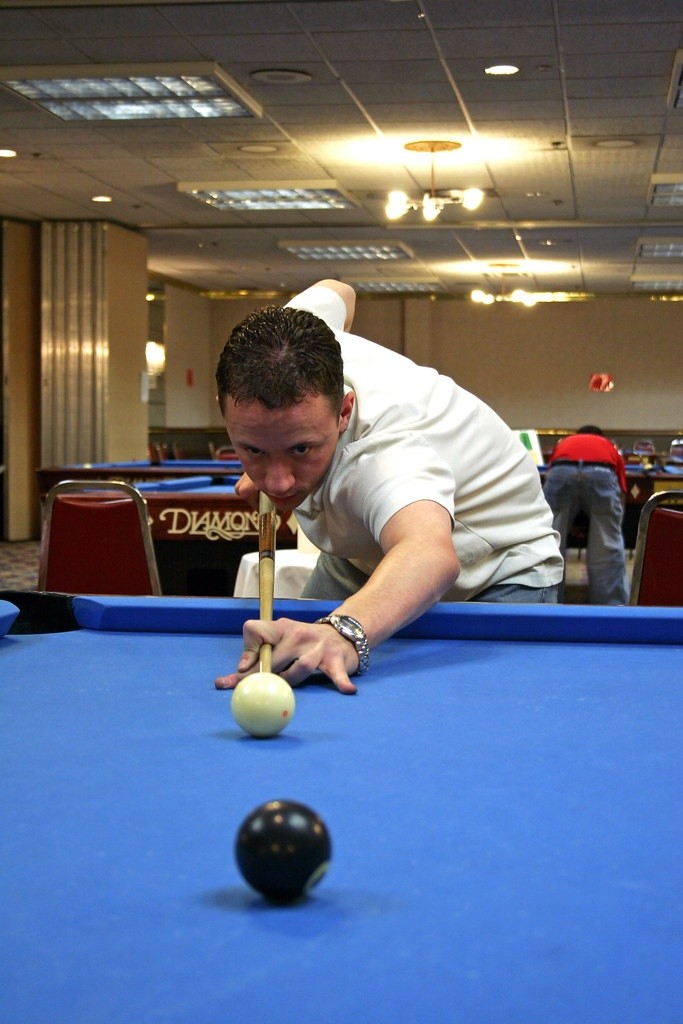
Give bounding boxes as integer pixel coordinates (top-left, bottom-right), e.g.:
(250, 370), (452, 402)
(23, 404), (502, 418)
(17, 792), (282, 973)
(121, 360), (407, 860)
(233, 799), (334, 901)
(231, 671), (296, 737)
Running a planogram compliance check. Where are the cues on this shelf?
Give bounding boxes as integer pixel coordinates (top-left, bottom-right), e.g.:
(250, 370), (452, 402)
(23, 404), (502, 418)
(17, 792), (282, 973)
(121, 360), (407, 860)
(258, 491), (277, 673)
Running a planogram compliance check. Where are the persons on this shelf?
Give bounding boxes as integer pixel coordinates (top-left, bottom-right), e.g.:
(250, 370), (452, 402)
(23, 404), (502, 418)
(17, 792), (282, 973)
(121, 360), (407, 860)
(215, 279), (563, 692)
(545, 424), (629, 606)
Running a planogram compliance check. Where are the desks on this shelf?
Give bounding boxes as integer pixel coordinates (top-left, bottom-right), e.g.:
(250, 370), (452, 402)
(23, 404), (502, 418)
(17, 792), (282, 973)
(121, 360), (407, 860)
(0, 589), (683, 1024)
(36, 450), (683, 561)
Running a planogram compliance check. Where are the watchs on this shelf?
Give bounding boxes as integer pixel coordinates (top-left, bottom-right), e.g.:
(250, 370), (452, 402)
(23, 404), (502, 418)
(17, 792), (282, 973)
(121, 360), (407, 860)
(315, 614), (369, 675)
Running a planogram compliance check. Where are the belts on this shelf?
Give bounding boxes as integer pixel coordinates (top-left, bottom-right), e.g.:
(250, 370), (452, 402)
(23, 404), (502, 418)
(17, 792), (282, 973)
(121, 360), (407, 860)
(550, 460), (614, 470)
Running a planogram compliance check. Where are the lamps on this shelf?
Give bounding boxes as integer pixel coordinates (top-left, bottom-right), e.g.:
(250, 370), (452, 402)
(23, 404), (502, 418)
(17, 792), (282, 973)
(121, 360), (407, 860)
(385, 141), (485, 221)
(468, 264), (539, 309)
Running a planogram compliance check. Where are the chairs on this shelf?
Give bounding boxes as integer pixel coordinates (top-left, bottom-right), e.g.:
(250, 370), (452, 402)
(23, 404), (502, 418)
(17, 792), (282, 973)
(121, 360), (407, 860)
(150, 442), (182, 461)
(38, 480), (159, 595)
(670, 440), (683, 456)
(633, 440), (654, 452)
(628, 489), (683, 607)
(208, 441), (239, 460)
